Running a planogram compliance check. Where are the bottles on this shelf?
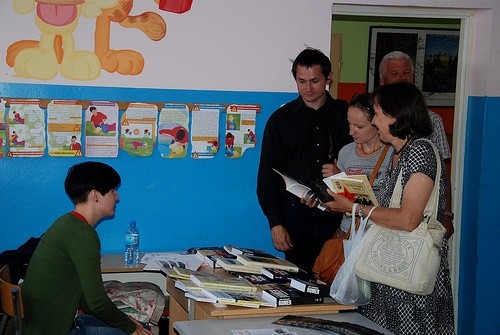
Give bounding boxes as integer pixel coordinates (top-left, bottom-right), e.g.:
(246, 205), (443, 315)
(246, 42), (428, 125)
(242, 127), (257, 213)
(124, 220), (140, 268)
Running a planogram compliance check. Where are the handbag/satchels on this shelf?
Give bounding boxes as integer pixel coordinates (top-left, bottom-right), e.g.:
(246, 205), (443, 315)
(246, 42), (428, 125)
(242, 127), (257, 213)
(352, 138), (446, 296)
(328, 204), (377, 307)
(312, 235), (347, 286)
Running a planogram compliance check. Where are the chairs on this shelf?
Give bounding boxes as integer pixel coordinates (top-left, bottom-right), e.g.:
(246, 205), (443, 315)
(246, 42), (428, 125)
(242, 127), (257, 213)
(0, 264), (24, 335)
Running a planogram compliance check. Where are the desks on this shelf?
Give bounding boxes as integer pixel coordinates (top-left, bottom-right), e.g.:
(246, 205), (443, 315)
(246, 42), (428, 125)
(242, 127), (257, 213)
(99, 254), (395, 335)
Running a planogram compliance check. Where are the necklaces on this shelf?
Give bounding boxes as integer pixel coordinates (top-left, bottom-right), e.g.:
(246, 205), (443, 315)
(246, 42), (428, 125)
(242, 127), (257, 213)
(361, 142), (384, 156)
(393, 137), (412, 156)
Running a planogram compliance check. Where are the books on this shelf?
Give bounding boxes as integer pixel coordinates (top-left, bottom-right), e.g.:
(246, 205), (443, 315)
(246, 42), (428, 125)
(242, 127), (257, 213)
(159, 247), (330, 310)
(272, 168), (376, 218)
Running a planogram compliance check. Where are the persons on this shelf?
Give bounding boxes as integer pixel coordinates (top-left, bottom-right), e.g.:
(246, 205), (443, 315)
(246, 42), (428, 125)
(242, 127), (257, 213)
(312, 92), (396, 286)
(379, 52), (455, 240)
(315, 83), (456, 335)
(256, 47), (354, 277)
(15, 161), (153, 335)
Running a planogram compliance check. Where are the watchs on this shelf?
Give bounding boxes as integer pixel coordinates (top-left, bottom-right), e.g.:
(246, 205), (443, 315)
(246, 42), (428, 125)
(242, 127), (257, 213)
(358, 205), (366, 219)
(444, 212), (455, 219)
